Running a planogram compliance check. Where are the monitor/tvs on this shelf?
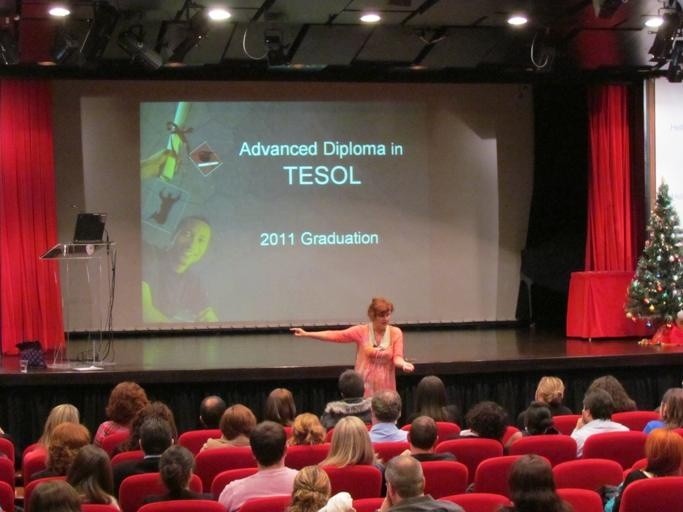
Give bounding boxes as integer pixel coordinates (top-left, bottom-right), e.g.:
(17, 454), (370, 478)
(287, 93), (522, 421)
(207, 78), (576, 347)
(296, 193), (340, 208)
(72, 213), (106, 244)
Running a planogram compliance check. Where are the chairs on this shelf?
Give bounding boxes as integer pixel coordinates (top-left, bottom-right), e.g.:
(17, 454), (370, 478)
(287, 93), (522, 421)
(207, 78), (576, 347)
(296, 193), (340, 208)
(0, 404), (682, 510)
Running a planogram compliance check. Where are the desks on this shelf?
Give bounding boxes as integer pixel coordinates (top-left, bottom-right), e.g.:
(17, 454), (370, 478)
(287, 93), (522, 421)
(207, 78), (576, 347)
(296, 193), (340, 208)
(566, 268), (649, 342)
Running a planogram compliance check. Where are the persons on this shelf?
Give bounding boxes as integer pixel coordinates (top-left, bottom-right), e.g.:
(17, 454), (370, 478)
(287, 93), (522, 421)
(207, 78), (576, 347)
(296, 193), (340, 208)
(141, 216), (218, 322)
(290, 295), (416, 399)
(140, 146), (181, 181)
